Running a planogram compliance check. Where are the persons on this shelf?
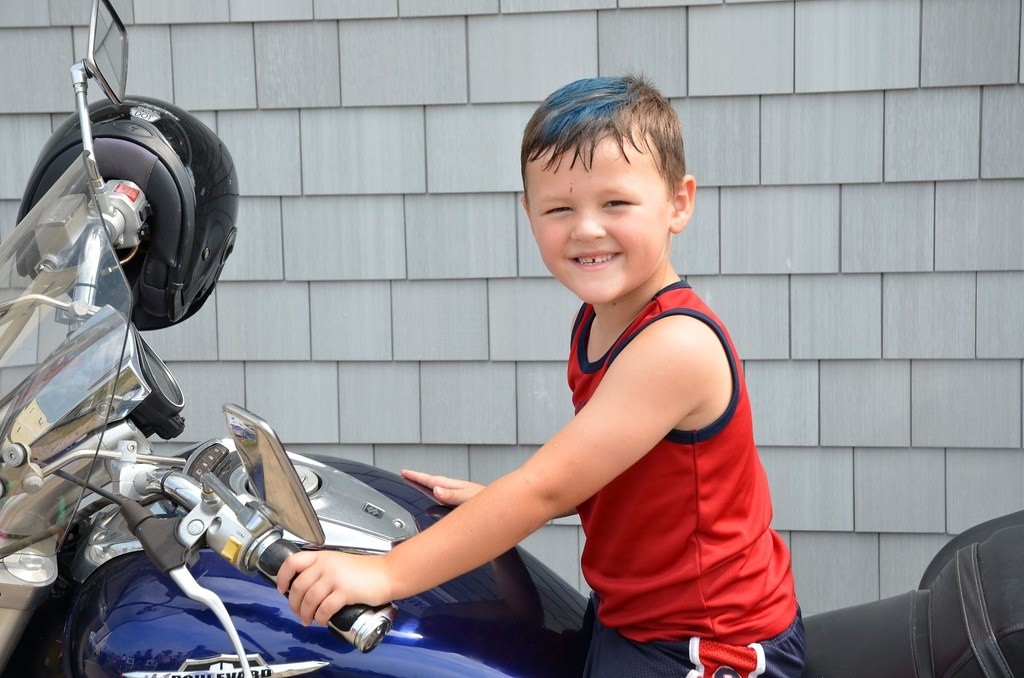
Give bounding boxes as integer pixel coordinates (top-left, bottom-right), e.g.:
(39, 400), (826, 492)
(272, 73), (810, 678)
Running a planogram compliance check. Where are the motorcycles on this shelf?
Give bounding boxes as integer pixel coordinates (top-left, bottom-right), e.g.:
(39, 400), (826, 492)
(1, 1), (1023, 678)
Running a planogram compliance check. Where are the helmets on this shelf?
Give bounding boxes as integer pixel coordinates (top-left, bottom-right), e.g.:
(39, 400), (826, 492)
(13, 96), (239, 331)
